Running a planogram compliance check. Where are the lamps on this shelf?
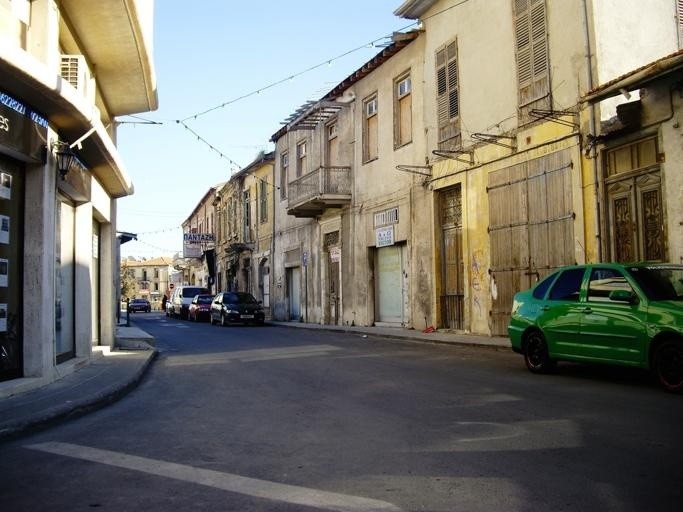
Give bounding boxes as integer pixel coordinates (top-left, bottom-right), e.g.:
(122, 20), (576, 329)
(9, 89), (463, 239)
(54, 143), (75, 180)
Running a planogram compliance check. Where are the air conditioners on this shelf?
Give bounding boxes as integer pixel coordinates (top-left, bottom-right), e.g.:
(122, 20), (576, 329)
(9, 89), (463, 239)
(59, 54), (97, 105)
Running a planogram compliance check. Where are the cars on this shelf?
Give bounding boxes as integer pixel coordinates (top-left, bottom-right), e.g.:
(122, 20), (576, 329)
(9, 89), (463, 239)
(128, 298), (151, 313)
(506, 239), (683, 393)
(164, 285), (265, 328)
